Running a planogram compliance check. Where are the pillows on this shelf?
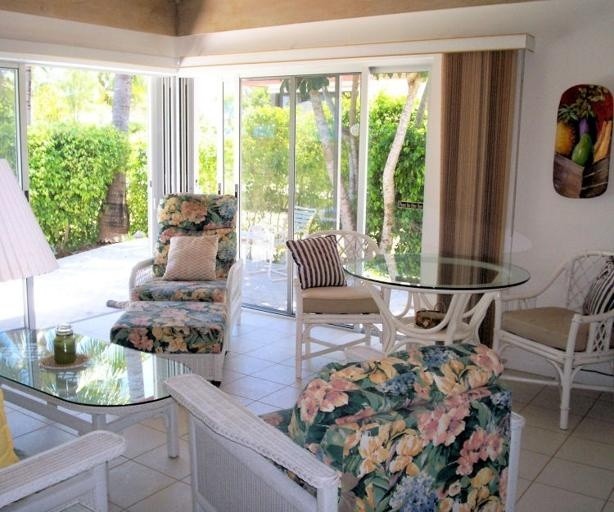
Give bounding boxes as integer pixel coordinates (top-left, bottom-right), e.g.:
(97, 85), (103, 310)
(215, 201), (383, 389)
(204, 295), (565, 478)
(160, 236), (222, 283)
(287, 235), (348, 289)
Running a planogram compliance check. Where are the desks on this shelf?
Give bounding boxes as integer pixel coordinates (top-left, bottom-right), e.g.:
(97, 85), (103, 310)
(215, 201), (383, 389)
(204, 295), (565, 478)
(341, 251), (529, 359)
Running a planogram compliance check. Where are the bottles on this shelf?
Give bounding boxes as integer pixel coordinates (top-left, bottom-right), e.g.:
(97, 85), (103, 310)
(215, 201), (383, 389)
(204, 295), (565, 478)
(53, 323), (76, 365)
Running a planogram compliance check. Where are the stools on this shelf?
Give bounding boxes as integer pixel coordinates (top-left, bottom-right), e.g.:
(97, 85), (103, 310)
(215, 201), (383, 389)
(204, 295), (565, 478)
(107, 297), (229, 388)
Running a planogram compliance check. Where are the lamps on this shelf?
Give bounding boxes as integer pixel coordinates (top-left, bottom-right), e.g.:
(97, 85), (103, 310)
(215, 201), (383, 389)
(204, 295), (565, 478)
(0, 155), (62, 286)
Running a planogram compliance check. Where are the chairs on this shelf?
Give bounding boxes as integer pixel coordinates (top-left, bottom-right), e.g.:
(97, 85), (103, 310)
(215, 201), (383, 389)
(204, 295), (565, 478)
(484, 250), (614, 431)
(174, 342), (524, 512)
(128, 193), (244, 323)
(278, 231), (386, 385)
(268, 205), (315, 280)
(1, 389), (129, 512)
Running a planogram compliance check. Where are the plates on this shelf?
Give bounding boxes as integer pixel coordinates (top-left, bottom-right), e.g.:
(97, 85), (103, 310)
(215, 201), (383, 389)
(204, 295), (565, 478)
(40, 353), (89, 370)
